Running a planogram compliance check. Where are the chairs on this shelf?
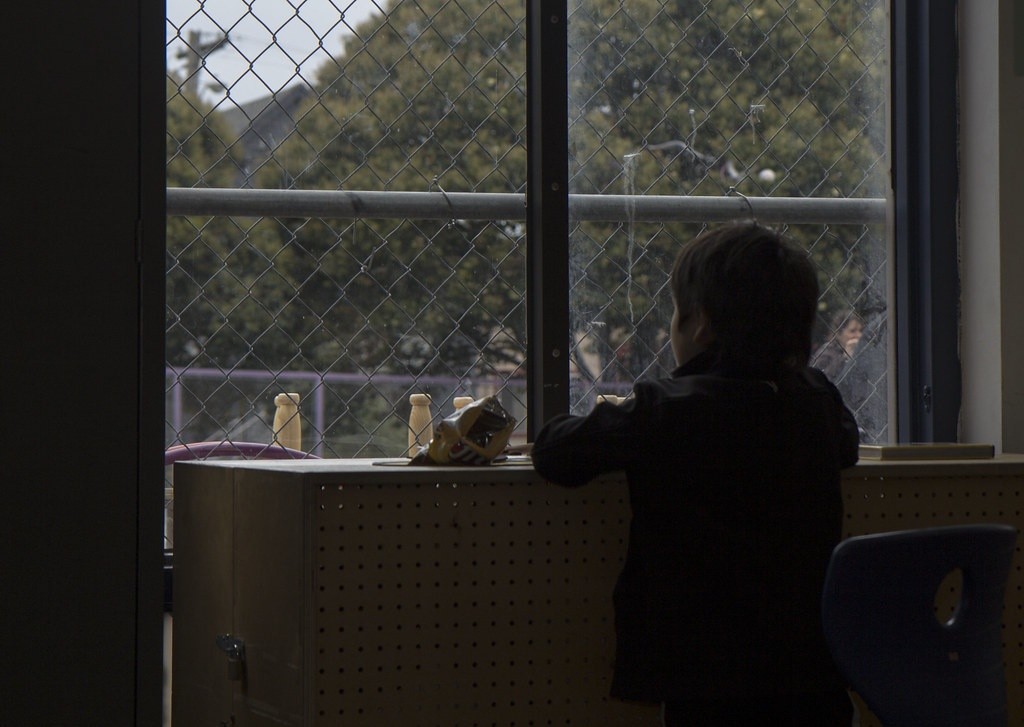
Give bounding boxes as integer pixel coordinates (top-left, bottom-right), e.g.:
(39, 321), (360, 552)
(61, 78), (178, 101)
(822, 523), (1017, 727)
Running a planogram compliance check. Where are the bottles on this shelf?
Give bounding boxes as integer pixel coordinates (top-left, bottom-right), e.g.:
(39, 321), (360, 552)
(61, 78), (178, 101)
(596, 393), (632, 407)
(408, 393), (432, 458)
(271, 393), (301, 452)
(452, 395), (474, 413)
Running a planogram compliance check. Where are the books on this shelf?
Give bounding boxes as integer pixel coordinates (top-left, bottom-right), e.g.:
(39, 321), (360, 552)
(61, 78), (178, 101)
(859, 442), (995, 461)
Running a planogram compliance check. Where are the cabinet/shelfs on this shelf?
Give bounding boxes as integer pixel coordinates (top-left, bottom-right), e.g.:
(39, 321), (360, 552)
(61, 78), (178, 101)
(172, 454), (1024, 727)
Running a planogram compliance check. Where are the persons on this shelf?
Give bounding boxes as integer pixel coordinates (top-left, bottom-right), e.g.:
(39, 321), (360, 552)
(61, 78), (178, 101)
(530, 222), (869, 727)
